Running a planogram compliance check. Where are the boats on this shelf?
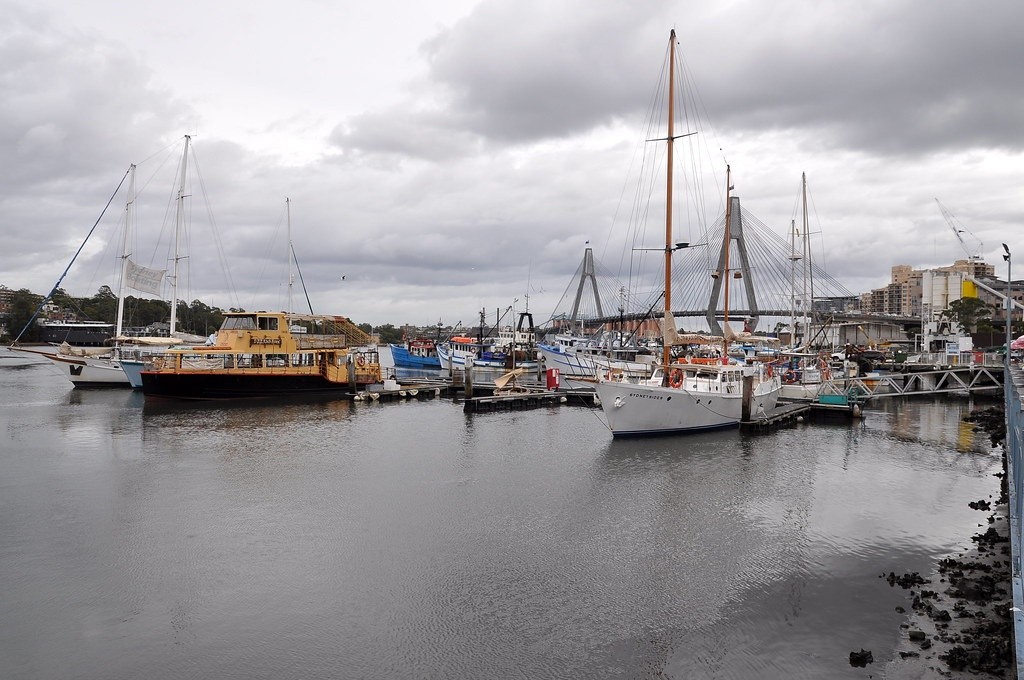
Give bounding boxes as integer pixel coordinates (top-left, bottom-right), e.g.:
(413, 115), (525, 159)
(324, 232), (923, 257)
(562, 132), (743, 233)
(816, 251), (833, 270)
(388, 305), (655, 378)
(140, 315), (381, 405)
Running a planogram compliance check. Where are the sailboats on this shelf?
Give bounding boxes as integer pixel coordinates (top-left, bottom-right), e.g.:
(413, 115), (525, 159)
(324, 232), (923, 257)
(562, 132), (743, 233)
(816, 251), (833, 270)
(102, 197), (318, 388)
(6, 133), (240, 388)
(564, 27), (905, 438)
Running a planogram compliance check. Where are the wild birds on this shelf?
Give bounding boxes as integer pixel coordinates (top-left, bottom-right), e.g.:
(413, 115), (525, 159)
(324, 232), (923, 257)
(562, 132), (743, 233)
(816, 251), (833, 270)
(342, 275), (345, 279)
(586, 239), (589, 244)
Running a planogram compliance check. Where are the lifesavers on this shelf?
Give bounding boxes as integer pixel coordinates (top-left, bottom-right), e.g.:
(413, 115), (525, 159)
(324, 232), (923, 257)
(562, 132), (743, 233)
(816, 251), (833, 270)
(470, 348), (476, 354)
(768, 364), (773, 377)
(357, 356), (365, 366)
(783, 370), (797, 383)
(389, 375), (397, 380)
(669, 368), (683, 388)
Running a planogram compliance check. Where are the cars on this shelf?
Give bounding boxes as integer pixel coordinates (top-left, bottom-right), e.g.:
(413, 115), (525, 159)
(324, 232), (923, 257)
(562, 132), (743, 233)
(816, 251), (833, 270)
(830, 348), (858, 361)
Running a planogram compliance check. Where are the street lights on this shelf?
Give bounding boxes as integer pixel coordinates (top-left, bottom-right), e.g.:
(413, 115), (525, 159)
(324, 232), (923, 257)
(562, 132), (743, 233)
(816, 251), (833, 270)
(1001, 243), (1011, 367)
